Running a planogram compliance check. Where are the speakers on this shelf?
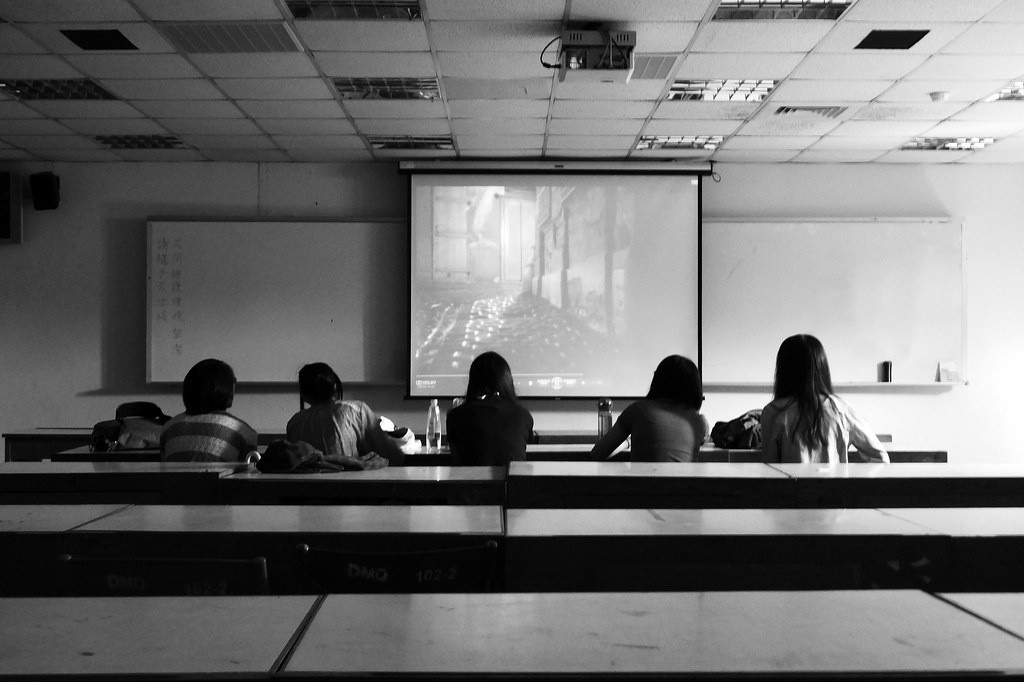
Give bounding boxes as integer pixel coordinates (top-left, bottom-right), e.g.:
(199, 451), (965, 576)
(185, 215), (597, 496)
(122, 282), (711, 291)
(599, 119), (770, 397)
(30, 172), (61, 210)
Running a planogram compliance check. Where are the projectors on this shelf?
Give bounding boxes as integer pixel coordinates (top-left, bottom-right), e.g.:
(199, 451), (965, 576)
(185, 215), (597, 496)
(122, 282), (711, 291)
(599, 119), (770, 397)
(558, 45), (635, 83)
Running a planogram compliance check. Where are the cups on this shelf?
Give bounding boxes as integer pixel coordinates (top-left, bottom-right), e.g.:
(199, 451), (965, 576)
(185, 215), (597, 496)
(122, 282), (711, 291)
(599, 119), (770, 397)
(597, 399), (613, 441)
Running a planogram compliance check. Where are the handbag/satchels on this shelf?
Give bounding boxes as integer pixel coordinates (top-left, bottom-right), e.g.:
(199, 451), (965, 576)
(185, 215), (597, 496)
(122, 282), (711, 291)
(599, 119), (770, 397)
(712, 410), (765, 449)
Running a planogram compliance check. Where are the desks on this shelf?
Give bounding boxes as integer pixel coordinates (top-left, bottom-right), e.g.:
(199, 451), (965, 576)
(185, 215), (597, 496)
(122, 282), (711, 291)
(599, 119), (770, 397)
(1, 425), (450, 461)
(876, 507), (1024, 590)
(504, 509), (950, 589)
(0, 461), (256, 504)
(524, 428), (949, 462)
(0, 503), (134, 596)
(933, 591), (1024, 639)
(275, 590), (1024, 681)
(0, 595), (326, 682)
(68, 503), (503, 595)
(507, 462), (792, 510)
(766, 462), (1023, 507)
(221, 465), (507, 504)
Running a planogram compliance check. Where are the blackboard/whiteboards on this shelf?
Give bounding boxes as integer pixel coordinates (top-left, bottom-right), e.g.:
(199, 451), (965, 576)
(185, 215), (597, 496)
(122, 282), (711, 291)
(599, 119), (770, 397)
(141, 213), (410, 392)
(699, 214), (965, 396)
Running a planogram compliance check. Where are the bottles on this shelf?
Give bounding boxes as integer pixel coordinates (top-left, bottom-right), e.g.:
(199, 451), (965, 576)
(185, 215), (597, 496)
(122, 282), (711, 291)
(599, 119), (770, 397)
(426, 399), (442, 454)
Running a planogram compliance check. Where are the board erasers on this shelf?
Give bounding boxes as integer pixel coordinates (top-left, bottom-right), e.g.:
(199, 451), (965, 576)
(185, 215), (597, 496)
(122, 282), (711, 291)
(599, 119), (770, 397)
(882, 361), (893, 382)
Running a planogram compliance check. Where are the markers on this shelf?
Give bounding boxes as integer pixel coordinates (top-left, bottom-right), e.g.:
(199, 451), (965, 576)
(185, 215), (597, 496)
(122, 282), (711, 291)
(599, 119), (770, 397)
(937, 361), (942, 382)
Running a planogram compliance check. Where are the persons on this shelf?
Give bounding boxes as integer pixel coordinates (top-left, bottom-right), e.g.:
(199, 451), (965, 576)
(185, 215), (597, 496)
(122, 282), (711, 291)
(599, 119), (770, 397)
(446, 351), (535, 465)
(761, 333), (893, 464)
(159, 358), (257, 463)
(588, 354), (710, 462)
(286, 362), (405, 462)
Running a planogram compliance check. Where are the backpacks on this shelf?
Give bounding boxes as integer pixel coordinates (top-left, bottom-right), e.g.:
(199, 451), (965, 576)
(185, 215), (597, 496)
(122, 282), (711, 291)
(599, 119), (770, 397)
(89, 415), (163, 451)
